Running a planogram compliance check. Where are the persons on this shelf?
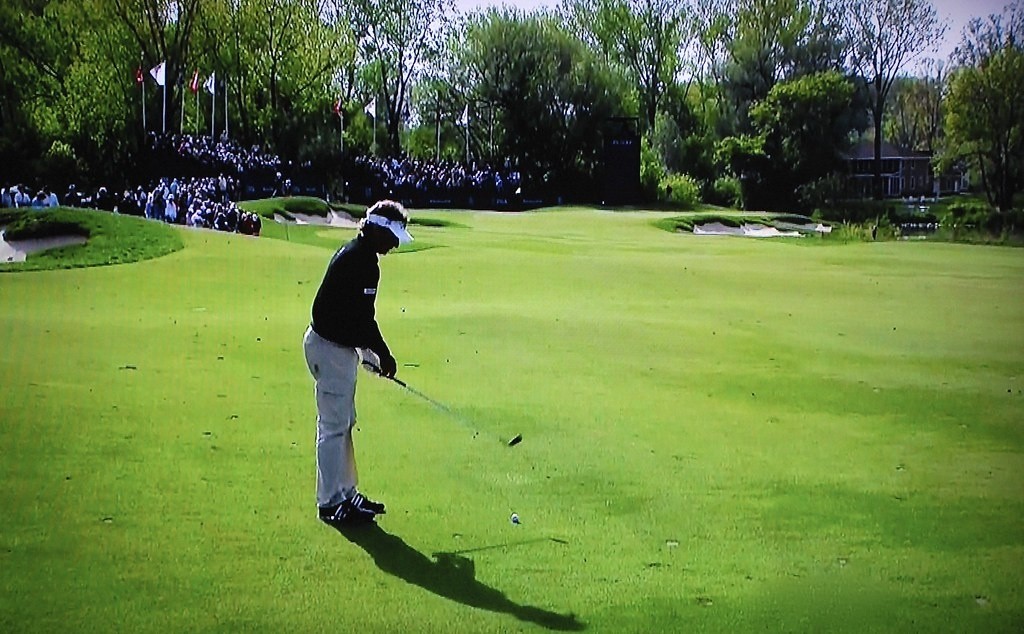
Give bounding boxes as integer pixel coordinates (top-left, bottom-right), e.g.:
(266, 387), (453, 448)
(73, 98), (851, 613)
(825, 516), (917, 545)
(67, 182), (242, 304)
(1, 176), (261, 236)
(302, 200), (417, 527)
(150, 128), (551, 209)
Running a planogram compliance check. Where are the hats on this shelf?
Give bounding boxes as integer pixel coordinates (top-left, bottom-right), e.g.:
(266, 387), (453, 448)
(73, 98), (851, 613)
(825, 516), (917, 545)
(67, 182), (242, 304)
(365, 212), (415, 243)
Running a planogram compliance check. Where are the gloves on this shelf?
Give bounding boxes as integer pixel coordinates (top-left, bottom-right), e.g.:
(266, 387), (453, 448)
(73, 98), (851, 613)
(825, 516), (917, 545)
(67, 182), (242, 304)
(361, 348), (380, 372)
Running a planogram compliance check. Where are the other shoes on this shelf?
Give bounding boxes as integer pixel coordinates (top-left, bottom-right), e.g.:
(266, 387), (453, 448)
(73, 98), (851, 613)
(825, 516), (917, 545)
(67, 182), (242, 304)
(347, 492), (384, 514)
(319, 499), (376, 524)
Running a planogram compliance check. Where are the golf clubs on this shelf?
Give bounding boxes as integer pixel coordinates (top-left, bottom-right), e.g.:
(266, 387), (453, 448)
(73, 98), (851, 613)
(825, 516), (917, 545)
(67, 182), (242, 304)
(511, 513), (519, 522)
(358, 357), (522, 447)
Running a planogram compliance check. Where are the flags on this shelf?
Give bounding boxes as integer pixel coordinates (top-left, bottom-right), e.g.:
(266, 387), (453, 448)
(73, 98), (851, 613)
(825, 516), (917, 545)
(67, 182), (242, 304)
(438, 91), (445, 127)
(214, 75), (227, 96)
(334, 92), (344, 116)
(189, 68), (200, 94)
(149, 60), (166, 86)
(363, 95), (377, 118)
(460, 104), (469, 128)
(177, 68), (185, 89)
(137, 57), (145, 85)
(400, 92), (410, 120)
(201, 72), (214, 95)
(489, 102), (497, 127)
